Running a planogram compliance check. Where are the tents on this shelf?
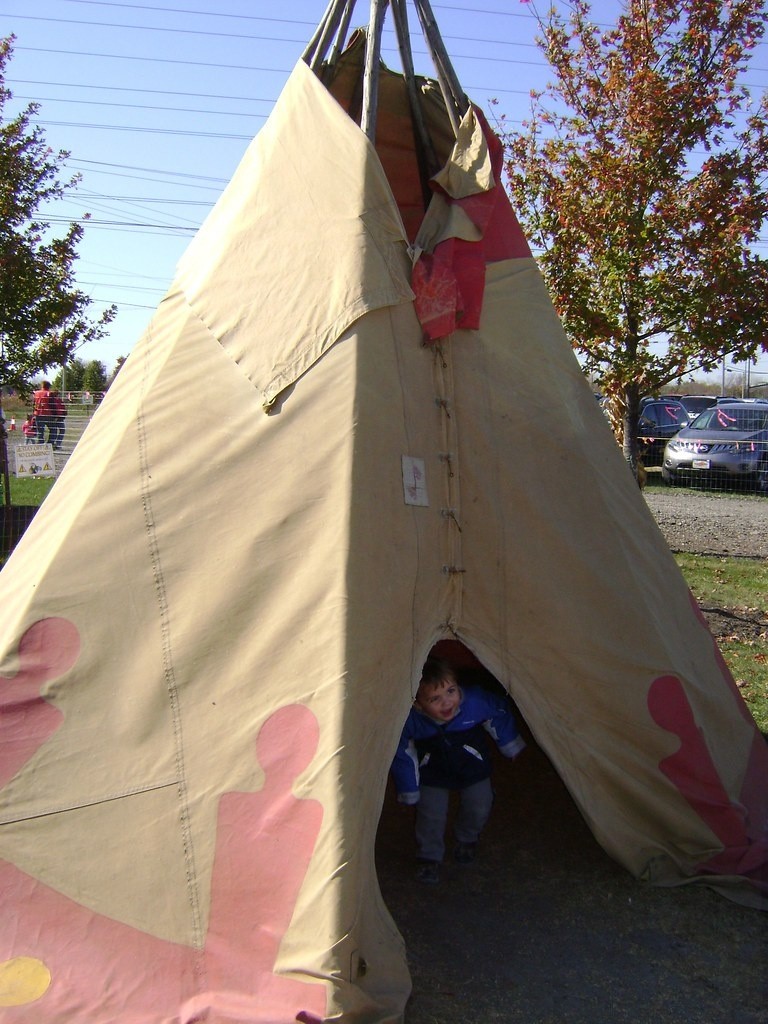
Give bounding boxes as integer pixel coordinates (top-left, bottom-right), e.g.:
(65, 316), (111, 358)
(0, 0), (768, 1024)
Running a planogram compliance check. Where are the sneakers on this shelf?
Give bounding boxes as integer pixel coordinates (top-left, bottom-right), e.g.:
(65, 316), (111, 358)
(453, 840), (476, 866)
(417, 861), (440, 885)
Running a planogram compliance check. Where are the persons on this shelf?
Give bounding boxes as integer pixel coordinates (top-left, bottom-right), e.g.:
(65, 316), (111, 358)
(392, 671), (526, 888)
(21, 381), (93, 450)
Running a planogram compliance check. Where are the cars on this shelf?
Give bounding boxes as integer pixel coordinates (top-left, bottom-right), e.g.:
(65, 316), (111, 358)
(661, 401), (768, 492)
(593, 389), (768, 463)
